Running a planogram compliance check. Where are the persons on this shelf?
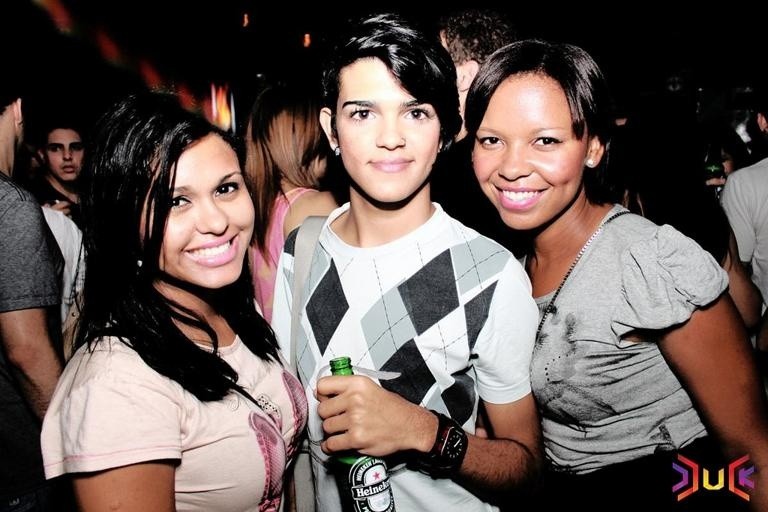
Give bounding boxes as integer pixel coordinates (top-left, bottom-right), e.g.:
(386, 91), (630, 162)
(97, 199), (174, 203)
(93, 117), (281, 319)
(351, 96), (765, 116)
(0, 9), (768, 512)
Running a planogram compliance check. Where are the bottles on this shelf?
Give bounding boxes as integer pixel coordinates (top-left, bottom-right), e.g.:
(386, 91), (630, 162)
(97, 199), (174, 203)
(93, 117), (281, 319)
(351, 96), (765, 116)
(324, 355), (396, 511)
